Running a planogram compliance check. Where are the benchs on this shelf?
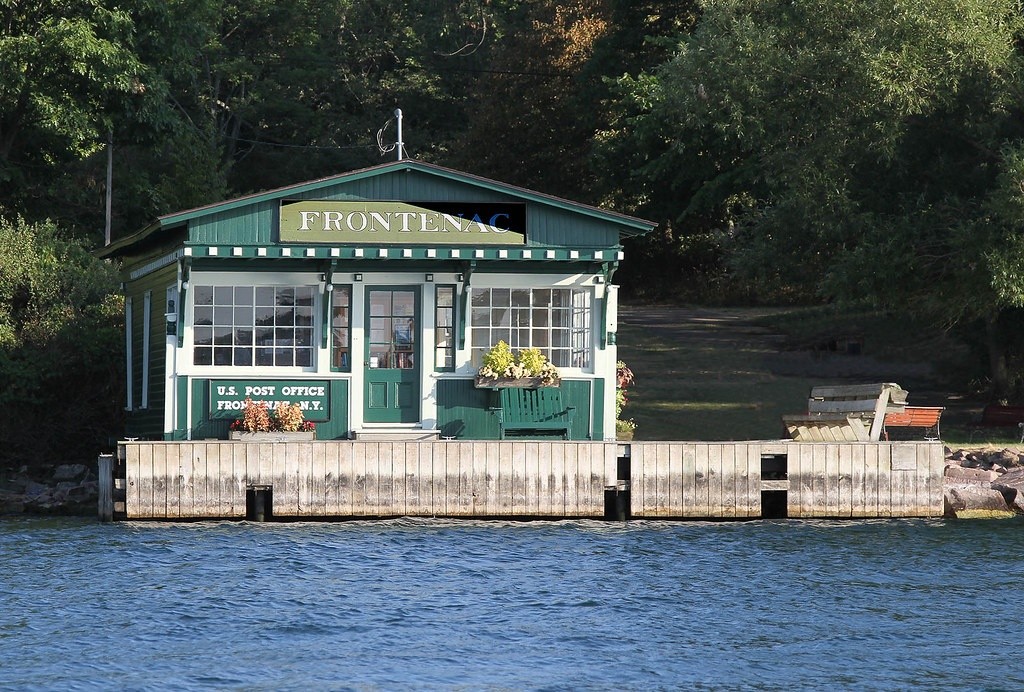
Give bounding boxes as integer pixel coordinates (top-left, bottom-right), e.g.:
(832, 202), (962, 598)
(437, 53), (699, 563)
(781, 385), (889, 442)
(882, 406), (946, 441)
(493, 387), (576, 441)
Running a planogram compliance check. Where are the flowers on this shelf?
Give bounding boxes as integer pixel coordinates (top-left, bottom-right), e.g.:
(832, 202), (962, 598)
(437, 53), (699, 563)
(616, 417), (637, 432)
(302, 421), (315, 432)
(230, 420), (243, 431)
(478, 361), (559, 386)
